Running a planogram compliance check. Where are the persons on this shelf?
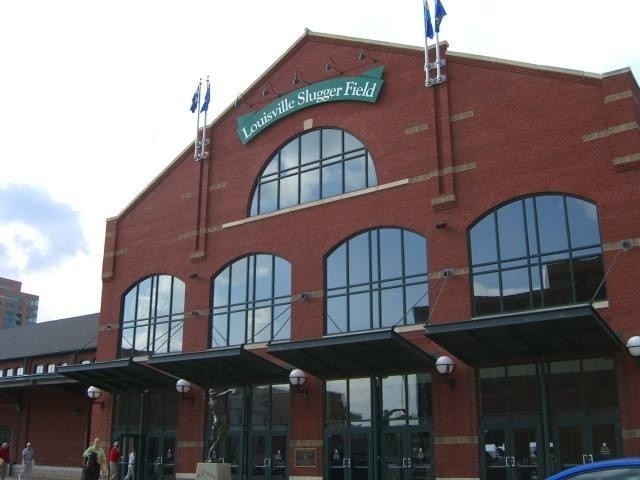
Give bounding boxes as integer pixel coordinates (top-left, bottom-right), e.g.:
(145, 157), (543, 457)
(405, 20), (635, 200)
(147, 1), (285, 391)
(19, 442), (35, 480)
(108, 441), (121, 480)
(82, 437), (106, 480)
(206, 387), (240, 463)
(124, 448), (137, 480)
(0, 442), (10, 480)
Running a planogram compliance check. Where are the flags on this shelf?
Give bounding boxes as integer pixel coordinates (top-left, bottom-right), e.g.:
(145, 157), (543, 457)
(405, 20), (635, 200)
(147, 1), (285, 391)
(434, 0), (447, 32)
(200, 83), (210, 113)
(190, 86), (199, 113)
(424, 3), (434, 39)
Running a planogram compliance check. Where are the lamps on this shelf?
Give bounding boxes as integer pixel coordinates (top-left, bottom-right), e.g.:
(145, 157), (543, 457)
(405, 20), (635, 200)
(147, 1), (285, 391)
(288, 368), (310, 401)
(434, 355), (457, 388)
(624, 336), (640, 366)
(88, 387), (106, 407)
(234, 47), (375, 109)
(176, 380), (195, 402)
